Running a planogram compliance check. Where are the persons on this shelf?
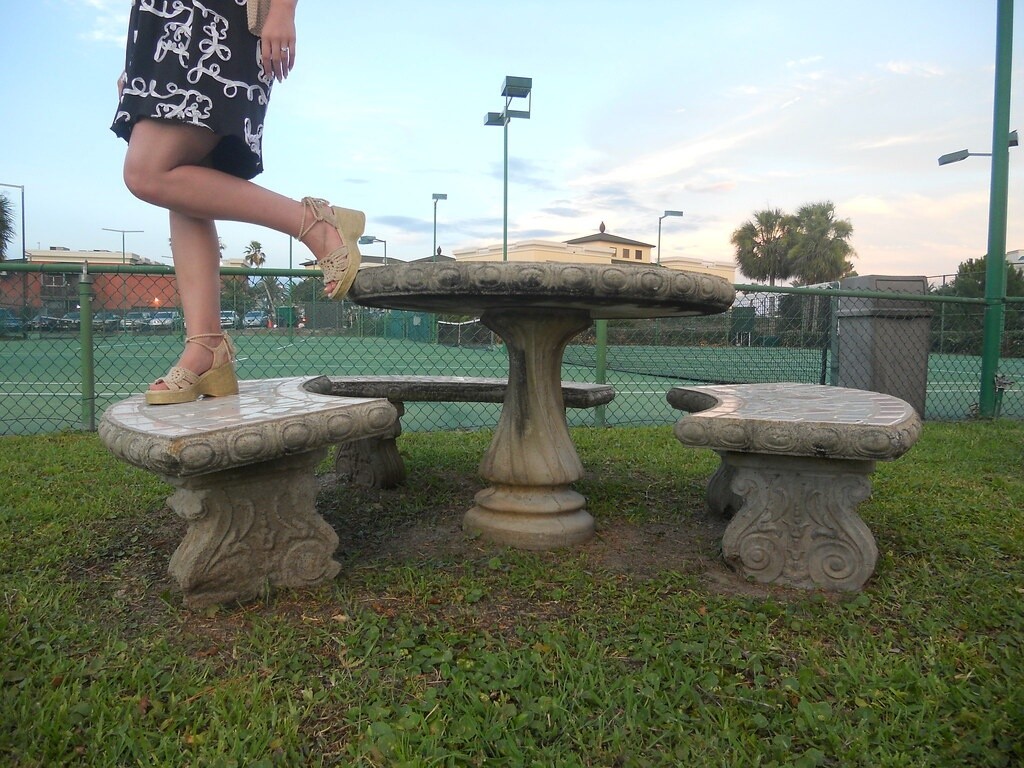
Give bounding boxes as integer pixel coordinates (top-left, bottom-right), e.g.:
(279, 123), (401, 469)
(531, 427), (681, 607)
(112, 0), (368, 408)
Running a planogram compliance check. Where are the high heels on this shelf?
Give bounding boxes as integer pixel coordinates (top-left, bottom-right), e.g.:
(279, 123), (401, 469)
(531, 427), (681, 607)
(144, 329), (240, 405)
(295, 196), (366, 303)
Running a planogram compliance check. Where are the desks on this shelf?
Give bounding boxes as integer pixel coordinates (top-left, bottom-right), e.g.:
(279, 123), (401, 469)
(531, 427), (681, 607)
(350, 266), (735, 552)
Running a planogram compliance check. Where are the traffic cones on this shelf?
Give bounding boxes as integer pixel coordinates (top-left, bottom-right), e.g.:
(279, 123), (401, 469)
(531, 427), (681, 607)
(267, 319), (273, 329)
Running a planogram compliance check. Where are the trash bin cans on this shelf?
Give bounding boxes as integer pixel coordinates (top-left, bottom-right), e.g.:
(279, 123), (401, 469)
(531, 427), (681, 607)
(836, 274), (932, 421)
(274, 305), (299, 327)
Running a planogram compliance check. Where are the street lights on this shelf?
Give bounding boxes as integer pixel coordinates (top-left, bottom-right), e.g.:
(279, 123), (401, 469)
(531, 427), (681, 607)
(484, 76), (532, 260)
(432, 193), (447, 262)
(102, 228), (144, 335)
(358, 236), (387, 266)
(657, 210), (683, 262)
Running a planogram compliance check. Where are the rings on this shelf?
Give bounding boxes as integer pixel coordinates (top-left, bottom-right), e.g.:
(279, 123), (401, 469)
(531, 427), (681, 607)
(280, 47), (288, 52)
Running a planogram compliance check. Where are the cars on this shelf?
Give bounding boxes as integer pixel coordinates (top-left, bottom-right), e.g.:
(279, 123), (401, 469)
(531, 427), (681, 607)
(142, 312), (151, 322)
(29, 315), (56, 331)
(244, 311), (272, 327)
(120, 312), (144, 331)
(148, 311), (181, 330)
(219, 310), (239, 328)
(92, 312), (117, 332)
(3, 318), (22, 332)
(113, 315), (121, 321)
(61, 312), (81, 330)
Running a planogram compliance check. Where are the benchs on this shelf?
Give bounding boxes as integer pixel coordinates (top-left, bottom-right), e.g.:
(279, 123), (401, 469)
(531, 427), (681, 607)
(666, 383), (923, 592)
(97, 375), (616, 614)
